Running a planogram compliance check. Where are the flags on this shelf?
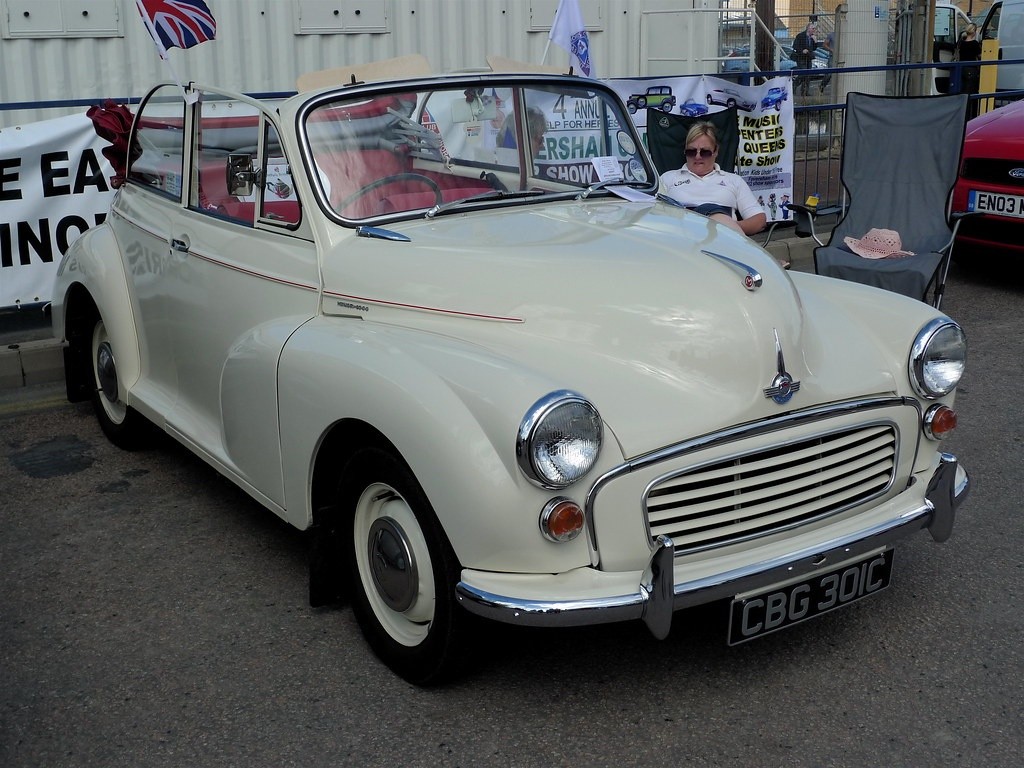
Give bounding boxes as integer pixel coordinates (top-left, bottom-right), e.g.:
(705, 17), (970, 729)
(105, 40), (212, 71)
(135, 0), (219, 59)
(549, 0), (602, 97)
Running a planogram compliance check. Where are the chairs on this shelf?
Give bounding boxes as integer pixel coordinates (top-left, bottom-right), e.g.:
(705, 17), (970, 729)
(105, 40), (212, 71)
(786, 92), (986, 311)
(373, 187), (499, 214)
(647, 102), (797, 268)
(217, 202), (301, 224)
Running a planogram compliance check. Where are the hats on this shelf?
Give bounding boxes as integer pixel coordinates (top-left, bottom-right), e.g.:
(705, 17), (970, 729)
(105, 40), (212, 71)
(844, 228), (917, 259)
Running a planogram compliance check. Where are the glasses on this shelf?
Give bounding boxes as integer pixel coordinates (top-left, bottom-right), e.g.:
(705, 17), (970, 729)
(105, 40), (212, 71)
(684, 148), (716, 159)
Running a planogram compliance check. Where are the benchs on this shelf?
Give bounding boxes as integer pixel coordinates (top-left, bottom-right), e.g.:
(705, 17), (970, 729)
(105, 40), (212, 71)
(194, 148), (410, 221)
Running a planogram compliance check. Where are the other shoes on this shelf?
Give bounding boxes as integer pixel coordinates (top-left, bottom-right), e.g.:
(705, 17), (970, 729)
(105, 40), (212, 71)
(801, 92), (811, 96)
(819, 85), (824, 93)
(793, 82), (796, 95)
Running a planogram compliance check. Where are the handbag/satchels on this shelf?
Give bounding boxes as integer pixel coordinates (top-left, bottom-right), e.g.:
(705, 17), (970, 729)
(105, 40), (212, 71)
(790, 51), (802, 63)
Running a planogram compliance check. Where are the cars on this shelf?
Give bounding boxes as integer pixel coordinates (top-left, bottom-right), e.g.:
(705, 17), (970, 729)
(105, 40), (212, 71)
(956, 96), (1024, 280)
(719, 37), (833, 80)
(50, 65), (969, 694)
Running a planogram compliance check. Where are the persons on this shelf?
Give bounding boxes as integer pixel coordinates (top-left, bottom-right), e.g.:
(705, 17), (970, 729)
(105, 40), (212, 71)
(951, 22), (984, 95)
(790, 24), (816, 96)
(818, 29), (837, 93)
(495, 109), (546, 160)
(653, 122), (792, 270)
